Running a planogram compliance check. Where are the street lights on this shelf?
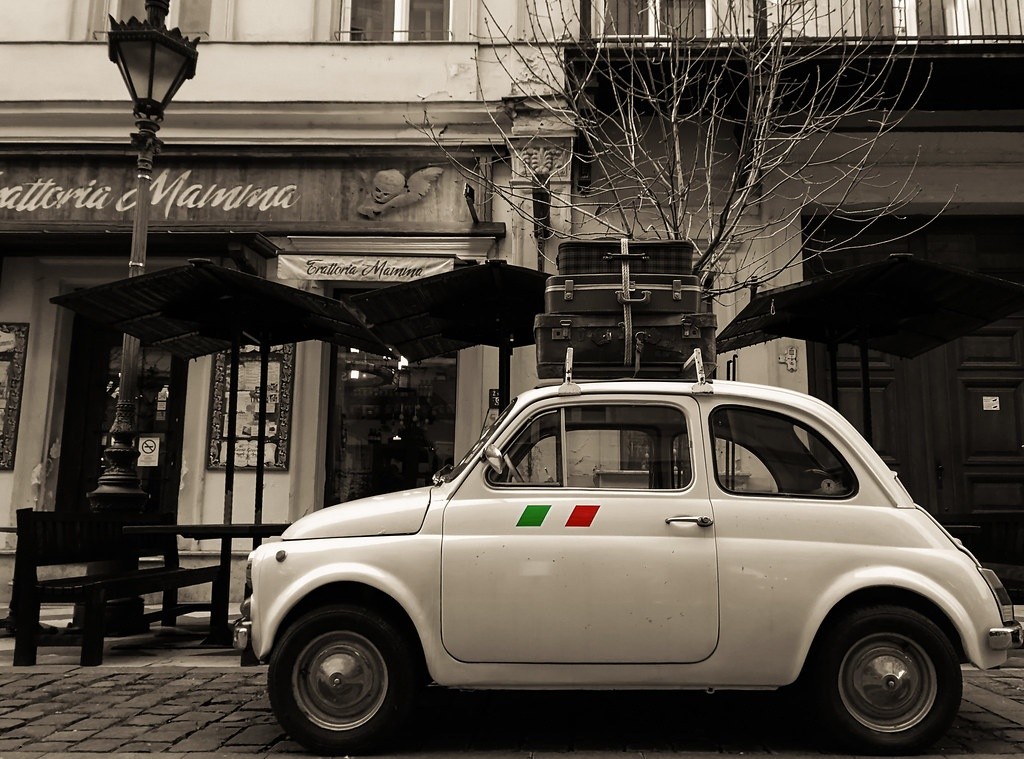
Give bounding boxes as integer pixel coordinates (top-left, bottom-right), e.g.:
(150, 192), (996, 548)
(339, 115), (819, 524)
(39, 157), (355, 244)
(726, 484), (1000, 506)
(65, 0), (210, 636)
(397, 367), (412, 492)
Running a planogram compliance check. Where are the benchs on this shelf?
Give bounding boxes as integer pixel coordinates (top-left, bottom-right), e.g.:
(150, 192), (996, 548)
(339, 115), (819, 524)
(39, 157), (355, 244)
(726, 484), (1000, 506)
(11, 507), (231, 666)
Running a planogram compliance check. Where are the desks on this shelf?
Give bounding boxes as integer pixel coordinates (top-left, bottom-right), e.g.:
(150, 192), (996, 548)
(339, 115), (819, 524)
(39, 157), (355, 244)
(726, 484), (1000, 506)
(122, 522), (292, 668)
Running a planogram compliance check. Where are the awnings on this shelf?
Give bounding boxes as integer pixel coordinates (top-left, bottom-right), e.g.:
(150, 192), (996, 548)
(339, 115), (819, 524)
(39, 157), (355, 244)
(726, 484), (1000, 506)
(276, 251), (469, 282)
(715, 254), (1023, 446)
(0, 229), (284, 273)
(49, 257), (400, 648)
(351, 261), (554, 417)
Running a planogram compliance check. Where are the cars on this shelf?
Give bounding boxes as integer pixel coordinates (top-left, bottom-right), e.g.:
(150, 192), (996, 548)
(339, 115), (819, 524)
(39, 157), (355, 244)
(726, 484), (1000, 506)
(236, 368), (1013, 757)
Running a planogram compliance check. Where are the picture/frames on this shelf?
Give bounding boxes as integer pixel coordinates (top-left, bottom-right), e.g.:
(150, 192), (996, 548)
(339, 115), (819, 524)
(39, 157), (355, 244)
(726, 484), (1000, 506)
(205, 340), (299, 472)
(0, 322), (29, 472)
